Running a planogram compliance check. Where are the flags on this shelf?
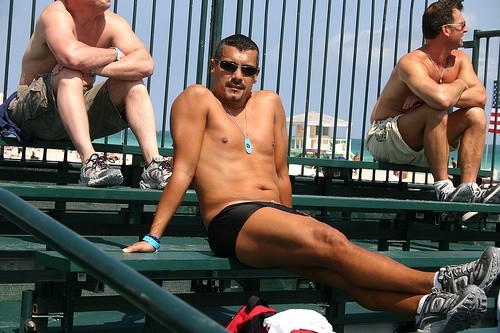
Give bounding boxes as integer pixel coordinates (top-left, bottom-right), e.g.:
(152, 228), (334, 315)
(488, 81), (500, 133)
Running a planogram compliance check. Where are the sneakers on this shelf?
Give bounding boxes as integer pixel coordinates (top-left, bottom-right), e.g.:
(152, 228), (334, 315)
(139, 155), (174, 190)
(78, 153), (123, 188)
(438, 183), (474, 222)
(438, 246), (500, 295)
(416, 284), (487, 333)
(462, 183), (500, 222)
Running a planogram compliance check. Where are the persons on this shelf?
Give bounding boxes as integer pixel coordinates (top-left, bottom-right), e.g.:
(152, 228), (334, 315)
(365, 0), (500, 225)
(124, 34), (500, 333)
(8, 0), (174, 189)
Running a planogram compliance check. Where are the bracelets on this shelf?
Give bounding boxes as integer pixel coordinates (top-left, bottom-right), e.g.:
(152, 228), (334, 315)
(142, 233), (160, 252)
(90, 69), (96, 79)
(111, 46), (122, 61)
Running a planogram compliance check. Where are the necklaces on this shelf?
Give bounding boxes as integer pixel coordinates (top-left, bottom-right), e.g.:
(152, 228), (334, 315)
(224, 103), (253, 154)
(425, 45), (451, 84)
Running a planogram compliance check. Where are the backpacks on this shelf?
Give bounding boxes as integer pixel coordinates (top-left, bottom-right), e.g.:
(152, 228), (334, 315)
(224, 295), (277, 333)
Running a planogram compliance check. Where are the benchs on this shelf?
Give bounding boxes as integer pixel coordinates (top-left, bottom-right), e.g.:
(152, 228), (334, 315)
(0, 135), (500, 333)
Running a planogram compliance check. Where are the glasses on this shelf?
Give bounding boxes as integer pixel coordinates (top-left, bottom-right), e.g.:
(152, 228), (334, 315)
(215, 59), (258, 76)
(438, 20), (466, 28)
